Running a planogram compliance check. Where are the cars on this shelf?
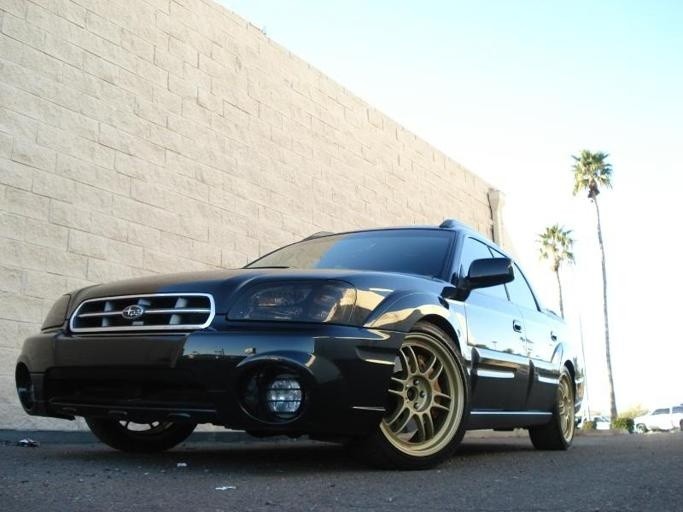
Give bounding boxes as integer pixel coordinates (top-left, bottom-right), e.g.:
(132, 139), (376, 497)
(16, 219), (584, 470)
(585, 415), (610, 430)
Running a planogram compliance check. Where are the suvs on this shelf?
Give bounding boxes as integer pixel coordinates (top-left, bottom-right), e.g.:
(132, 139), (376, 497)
(633, 404), (682, 434)
